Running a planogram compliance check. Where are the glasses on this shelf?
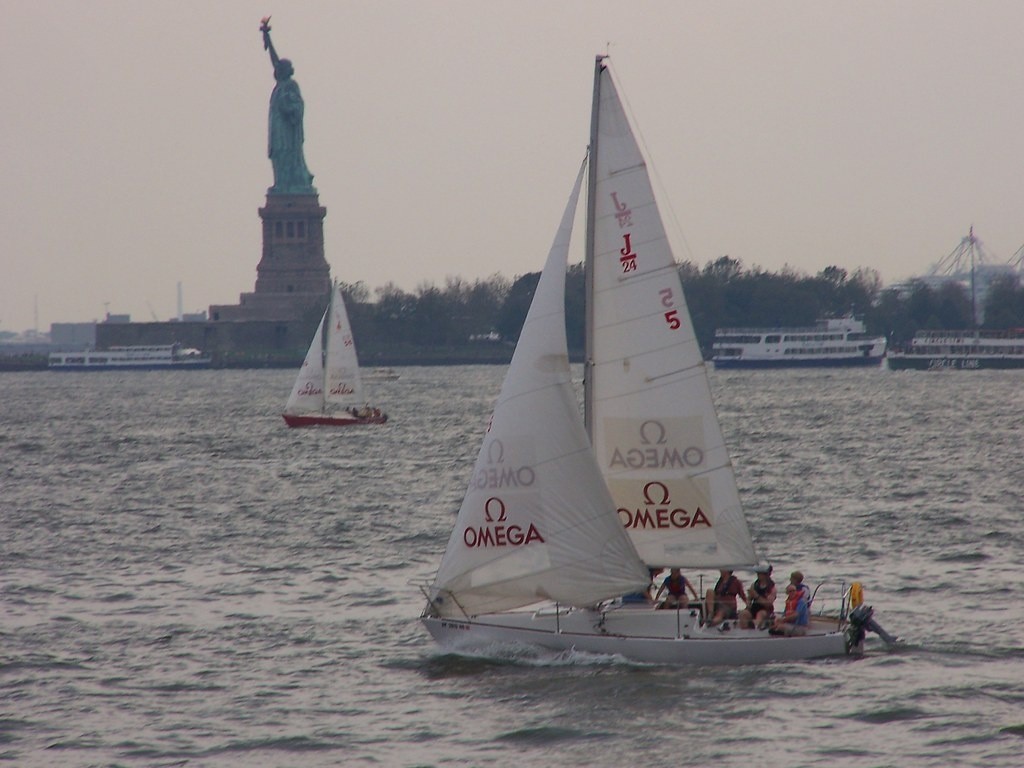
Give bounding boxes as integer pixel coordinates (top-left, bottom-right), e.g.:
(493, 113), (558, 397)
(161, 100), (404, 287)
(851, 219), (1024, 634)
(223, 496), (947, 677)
(788, 590), (795, 592)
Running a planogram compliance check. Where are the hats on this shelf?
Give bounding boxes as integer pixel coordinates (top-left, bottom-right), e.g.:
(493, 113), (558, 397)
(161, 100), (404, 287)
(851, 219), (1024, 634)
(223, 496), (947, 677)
(752, 561), (772, 573)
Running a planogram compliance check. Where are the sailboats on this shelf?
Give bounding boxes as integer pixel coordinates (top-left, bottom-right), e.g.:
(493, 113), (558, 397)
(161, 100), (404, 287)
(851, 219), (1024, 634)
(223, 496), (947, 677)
(280, 276), (388, 427)
(417, 53), (899, 665)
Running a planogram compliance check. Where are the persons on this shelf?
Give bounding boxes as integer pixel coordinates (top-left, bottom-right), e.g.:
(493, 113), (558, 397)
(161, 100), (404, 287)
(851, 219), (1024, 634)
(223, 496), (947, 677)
(344, 406), (382, 418)
(265, 32), (316, 195)
(641, 566), (811, 635)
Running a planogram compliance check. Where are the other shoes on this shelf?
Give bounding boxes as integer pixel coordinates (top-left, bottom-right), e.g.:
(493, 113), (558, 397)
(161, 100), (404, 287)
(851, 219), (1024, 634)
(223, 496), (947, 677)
(748, 620), (757, 629)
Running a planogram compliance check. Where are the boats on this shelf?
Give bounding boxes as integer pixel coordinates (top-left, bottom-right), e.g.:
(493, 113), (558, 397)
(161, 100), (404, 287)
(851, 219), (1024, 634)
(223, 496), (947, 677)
(49, 342), (212, 370)
(712, 318), (887, 370)
(886, 329), (1024, 369)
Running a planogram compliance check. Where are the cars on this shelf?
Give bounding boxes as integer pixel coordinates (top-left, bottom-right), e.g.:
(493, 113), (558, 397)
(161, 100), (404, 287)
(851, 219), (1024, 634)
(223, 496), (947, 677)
(469, 327), (501, 341)
